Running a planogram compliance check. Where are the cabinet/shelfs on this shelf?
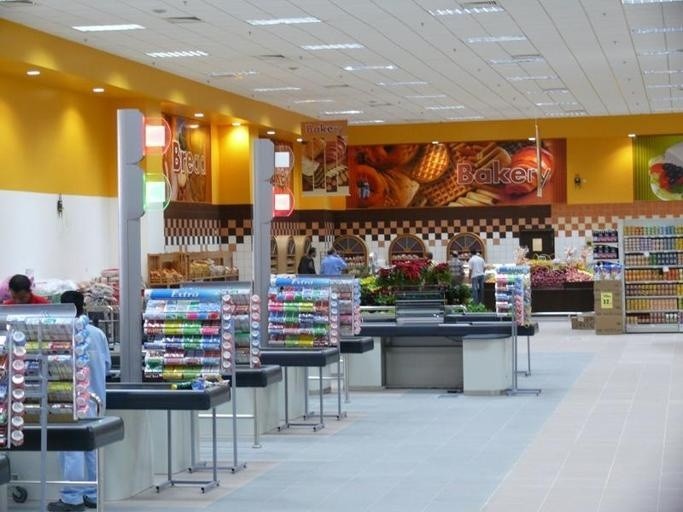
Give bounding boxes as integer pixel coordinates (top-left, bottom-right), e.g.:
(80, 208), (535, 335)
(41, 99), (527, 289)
(2, 299), (80, 424)
(494, 263), (531, 328)
(140, 281), (255, 384)
(266, 272), (358, 351)
(591, 218), (683, 335)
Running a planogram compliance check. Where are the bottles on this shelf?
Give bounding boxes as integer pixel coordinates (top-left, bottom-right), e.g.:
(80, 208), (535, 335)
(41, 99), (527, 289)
(590, 245), (619, 258)
(593, 230), (618, 243)
(594, 260), (622, 279)
(620, 225), (683, 325)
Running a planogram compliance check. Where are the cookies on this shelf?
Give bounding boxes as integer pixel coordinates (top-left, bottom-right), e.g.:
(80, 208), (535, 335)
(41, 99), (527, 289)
(384, 141), (512, 208)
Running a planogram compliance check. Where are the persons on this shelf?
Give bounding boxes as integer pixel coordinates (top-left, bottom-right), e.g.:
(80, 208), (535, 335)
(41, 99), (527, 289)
(48, 291), (111, 512)
(447, 250), (465, 281)
(467, 249), (487, 304)
(320, 248), (348, 276)
(298, 247), (317, 274)
(3, 274), (48, 304)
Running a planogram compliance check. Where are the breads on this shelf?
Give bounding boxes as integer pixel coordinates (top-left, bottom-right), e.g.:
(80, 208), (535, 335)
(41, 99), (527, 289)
(504, 145), (554, 195)
(302, 139), (349, 192)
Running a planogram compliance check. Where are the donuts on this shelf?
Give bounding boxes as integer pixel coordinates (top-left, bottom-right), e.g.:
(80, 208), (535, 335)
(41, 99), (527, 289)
(347, 143), (402, 208)
